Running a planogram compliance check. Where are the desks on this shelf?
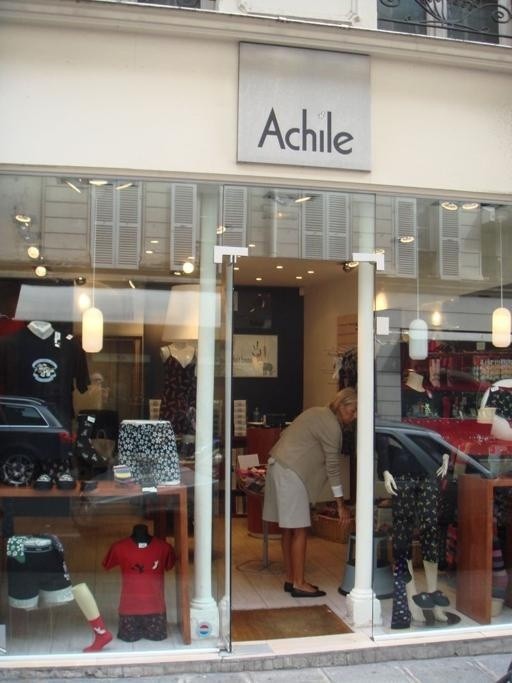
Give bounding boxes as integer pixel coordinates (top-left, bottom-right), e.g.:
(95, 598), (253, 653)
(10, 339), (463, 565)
(457, 472), (511, 624)
(1, 480), (191, 642)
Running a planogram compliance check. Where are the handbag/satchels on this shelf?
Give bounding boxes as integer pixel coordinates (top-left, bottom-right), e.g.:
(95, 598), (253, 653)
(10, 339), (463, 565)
(89, 428), (115, 463)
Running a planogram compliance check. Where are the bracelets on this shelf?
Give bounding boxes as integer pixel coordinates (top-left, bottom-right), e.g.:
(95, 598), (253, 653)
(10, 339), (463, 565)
(335, 497), (345, 504)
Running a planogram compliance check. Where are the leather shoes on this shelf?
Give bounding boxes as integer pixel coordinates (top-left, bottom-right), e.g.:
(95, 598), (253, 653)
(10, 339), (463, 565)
(282, 581), (320, 594)
(291, 586), (328, 599)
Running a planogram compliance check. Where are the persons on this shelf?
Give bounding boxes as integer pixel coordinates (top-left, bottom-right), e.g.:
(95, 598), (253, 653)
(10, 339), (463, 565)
(260, 387), (358, 599)
(5, 531), (75, 610)
(471, 374), (512, 610)
(375, 369), (451, 625)
(158, 332), (199, 460)
(117, 417), (186, 489)
(100, 523), (179, 641)
(69, 581), (114, 653)
(1, 317), (92, 445)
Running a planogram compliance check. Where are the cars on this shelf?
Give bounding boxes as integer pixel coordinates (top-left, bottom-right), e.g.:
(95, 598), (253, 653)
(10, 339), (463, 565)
(1, 396), (77, 487)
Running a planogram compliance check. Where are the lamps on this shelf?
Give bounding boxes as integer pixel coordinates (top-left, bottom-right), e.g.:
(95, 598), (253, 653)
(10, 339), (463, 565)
(490, 213), (512, 349)
(81, 181), (104, 353)
(408, 210), (428, 360)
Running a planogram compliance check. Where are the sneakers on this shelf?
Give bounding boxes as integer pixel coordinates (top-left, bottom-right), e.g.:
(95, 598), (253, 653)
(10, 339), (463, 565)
(54, 456), (76, 490)
(32, 459), (53, 489)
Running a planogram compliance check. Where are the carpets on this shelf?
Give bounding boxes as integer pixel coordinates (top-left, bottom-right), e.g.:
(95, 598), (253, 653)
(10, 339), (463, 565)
(230, 604), (353, 643)
